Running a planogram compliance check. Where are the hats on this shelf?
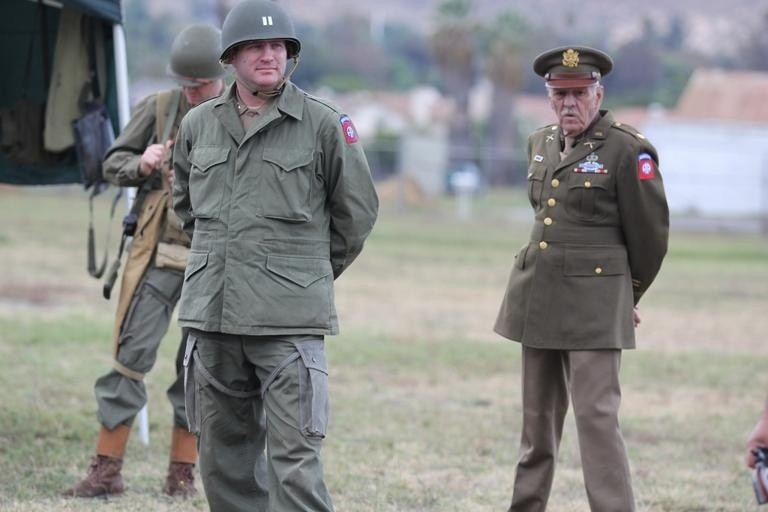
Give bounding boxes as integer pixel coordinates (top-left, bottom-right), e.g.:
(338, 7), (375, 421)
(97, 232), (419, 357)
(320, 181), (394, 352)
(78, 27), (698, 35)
(532, 45), (614, 89)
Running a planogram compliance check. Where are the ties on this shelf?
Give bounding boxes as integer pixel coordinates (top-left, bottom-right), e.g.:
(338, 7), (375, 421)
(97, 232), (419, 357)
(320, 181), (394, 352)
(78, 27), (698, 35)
(562, 136), (575, 156)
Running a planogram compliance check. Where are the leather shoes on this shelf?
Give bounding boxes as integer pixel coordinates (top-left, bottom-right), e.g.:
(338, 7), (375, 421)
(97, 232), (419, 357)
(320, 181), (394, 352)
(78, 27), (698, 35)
(60, 453), (125, 500)
(161, 461), (200, 501)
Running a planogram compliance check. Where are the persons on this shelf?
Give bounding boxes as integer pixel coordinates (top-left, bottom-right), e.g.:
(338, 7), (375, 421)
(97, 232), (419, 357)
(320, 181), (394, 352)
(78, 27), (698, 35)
(744, 387), (766, 508)
(166, 0), (385, 511)
(489, 43), (673, 512)
(55, 10), (235, 503)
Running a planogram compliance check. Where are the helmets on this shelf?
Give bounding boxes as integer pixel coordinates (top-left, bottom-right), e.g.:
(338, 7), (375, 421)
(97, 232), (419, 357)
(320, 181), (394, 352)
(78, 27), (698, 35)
(218, 0), (302, 66)
(165, 22), (235, 89)
